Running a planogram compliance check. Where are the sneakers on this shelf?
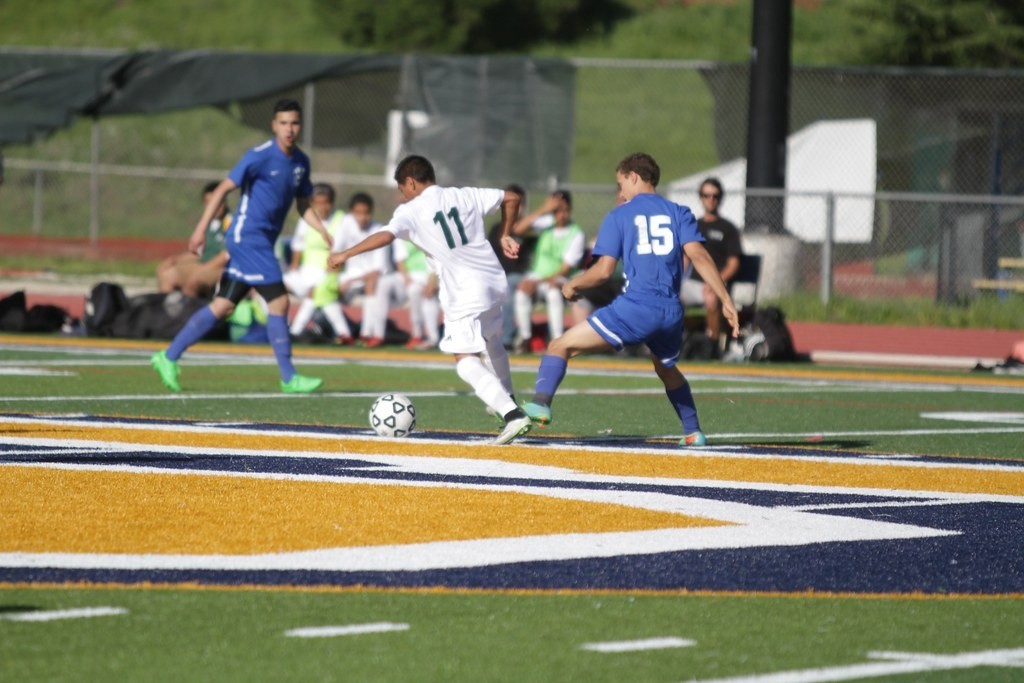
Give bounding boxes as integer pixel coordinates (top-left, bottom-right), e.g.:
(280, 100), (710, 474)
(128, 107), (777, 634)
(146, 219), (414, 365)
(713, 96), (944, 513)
(493, 415), (533, 444)
(151, 350), (181, 392)
(678, 431), (705, 446)
(279, 373), (322, 392)
(494, 400), (552, 428)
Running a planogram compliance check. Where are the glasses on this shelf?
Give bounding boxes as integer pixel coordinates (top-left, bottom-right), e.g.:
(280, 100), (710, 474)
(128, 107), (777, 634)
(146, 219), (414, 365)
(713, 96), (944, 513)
(702, 193), (720, 199)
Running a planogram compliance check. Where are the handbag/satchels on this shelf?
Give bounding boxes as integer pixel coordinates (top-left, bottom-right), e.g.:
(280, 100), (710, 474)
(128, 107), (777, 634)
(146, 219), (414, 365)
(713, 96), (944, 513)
(679, 331), (712, 359)
(744, 307), (793, 360)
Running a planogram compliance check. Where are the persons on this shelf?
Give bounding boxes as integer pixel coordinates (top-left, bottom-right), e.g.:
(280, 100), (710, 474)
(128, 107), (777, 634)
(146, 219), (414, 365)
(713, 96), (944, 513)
(327, 154), (532, 445)
(678, 178), (743, 355)
(487, 185), (538, 350)
(358, 237), (443, 350)
(513, 189), (585, 355)
(155, 181), (284, 300)
(313, 191), (392, 344)
(572, 190), (634, 326)
(283, 183), (345, 344)
(518, 152), (740, 446)
(150, 99), (329, 392)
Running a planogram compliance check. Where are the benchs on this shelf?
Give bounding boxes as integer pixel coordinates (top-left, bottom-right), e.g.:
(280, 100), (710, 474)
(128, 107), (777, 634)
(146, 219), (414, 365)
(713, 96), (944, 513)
(521, 234), (760, 359)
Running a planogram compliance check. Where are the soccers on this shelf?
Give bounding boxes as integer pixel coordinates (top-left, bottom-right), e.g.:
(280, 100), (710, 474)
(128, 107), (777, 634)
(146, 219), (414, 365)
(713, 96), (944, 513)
(368, 392), (419, 440)
